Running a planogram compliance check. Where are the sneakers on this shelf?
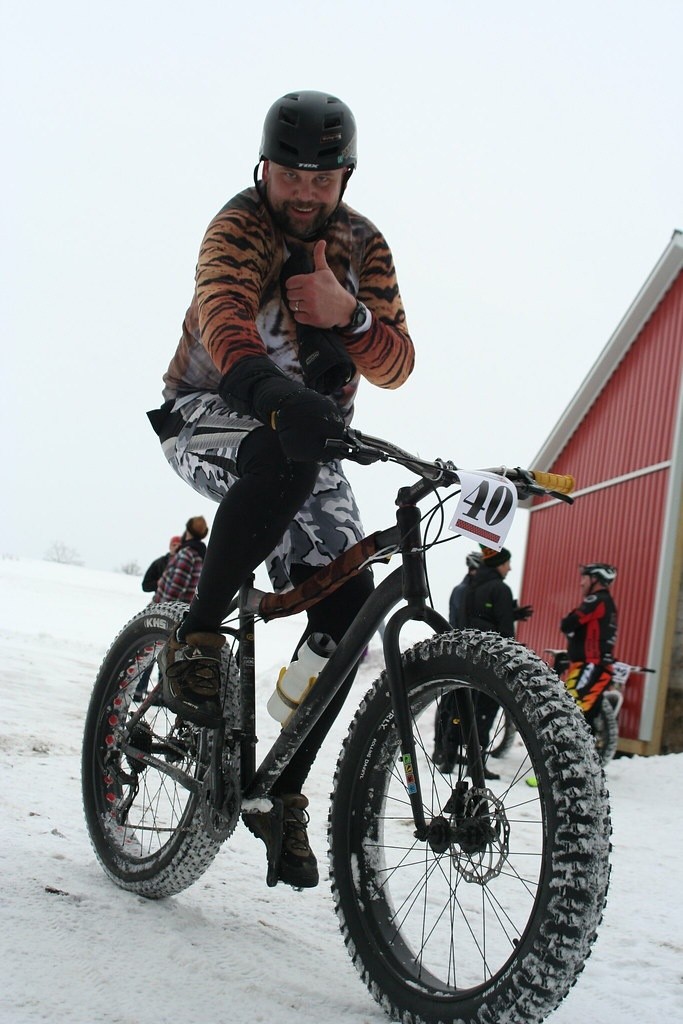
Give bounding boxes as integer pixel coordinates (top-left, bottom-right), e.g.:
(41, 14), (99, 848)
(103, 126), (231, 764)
(157, 611), (226, 729)
(242, 794), (318, 888)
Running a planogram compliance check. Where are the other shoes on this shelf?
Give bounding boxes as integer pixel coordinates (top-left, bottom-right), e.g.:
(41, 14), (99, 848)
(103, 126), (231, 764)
(526, 777), (538, 787)
(466, 766), (500, 779)
(133, 694), (144, 702)
(439, 762), (452, 774)
(432, 752), (468, 765)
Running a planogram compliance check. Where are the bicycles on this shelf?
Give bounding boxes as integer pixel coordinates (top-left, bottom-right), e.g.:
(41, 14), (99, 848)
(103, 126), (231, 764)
(486, 648), (658, 769)
(80, 415), (614, 1024)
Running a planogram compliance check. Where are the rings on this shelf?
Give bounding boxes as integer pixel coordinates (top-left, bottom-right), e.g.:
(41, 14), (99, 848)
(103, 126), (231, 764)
(293, 300), (300, 312)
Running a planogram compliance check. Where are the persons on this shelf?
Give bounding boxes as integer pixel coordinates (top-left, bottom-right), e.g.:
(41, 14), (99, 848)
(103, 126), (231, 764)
(429, 542), (533, 780)
(143, 87), (417, 890)
(132, 516), (208, 701)
(526, 563), (619, 785)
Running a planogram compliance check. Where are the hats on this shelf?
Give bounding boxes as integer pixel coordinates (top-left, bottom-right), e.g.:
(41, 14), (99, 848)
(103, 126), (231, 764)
(171, 537), (182, 545)
(187, 517), (208, 539)
(479, 543), (511, 567)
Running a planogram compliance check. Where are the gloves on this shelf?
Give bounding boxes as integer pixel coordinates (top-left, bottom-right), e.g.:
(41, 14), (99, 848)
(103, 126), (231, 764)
(513, 604), (534, 621)
(279, 247), (357, 396)
(217, 355), (345, 463)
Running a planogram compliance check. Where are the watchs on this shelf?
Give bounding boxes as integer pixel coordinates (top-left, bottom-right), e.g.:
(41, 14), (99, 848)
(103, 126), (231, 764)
(333, 297), (367, 335)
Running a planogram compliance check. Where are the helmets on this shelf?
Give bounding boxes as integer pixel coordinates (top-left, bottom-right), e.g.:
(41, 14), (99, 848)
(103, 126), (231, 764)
(259, 91), (357, 171)
(579, 563), (617, 586)
(466, 552), (482, 568)
